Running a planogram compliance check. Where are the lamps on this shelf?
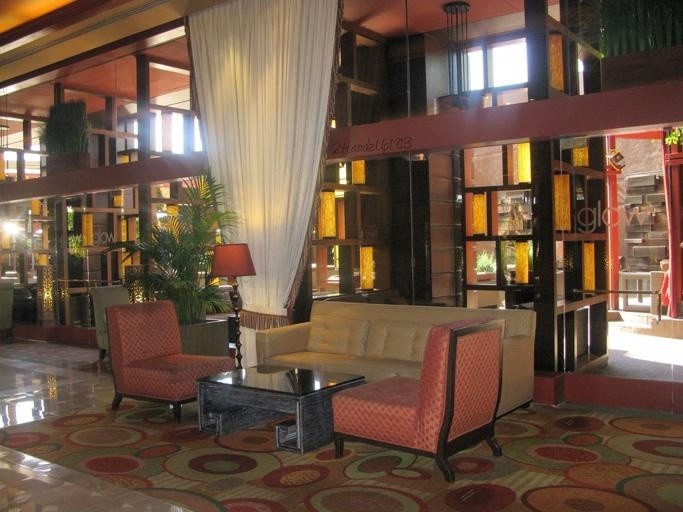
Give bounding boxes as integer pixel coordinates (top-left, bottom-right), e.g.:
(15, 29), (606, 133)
(436, 2), (485, 116)
(210, 241), (256, 369)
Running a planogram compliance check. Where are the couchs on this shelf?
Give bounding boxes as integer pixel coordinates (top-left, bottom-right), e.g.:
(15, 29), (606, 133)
(253, 299), (539, 485)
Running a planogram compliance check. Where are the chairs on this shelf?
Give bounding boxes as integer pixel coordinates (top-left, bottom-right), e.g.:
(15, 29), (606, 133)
(0, 282), (15, 343)
(331, 317), (509, 482)
(87, 285), (234, 424)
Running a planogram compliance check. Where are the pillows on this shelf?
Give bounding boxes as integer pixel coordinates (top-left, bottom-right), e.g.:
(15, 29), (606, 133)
(305, 314), (430, 364)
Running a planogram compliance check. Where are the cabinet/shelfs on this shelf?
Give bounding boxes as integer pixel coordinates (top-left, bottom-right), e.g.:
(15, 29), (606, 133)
(457, 1), (610, 380)
(0, 52), (202, 325)
(306, 28), (394, 302)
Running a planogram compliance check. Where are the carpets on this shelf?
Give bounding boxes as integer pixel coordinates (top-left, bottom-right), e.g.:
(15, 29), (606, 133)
(0, 394), (682, 511)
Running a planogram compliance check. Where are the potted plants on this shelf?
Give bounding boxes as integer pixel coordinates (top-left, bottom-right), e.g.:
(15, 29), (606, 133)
(102, 166), (242, 360)
